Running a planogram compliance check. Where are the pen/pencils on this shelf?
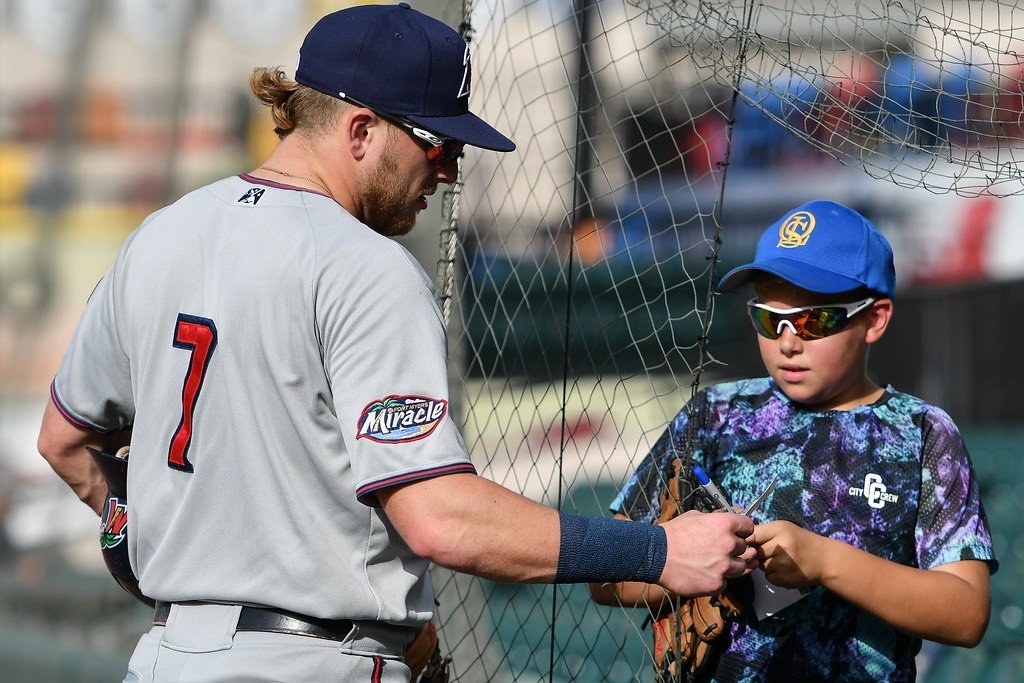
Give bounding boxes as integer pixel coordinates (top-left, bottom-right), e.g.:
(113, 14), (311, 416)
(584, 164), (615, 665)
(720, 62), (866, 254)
(692, 465), (738, 515)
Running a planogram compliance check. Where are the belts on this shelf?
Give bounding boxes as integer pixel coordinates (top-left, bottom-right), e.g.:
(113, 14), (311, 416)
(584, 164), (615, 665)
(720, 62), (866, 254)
(151, 600), (351, 642)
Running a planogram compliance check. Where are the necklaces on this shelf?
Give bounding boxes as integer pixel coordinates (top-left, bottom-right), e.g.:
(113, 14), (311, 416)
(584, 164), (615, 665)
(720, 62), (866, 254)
(257, 165), (333, 198)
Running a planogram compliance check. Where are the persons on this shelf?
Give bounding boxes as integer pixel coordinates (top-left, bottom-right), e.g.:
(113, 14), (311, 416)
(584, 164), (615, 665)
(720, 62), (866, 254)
(588, 200), (1000, 683)
(38, 3), (754, 683)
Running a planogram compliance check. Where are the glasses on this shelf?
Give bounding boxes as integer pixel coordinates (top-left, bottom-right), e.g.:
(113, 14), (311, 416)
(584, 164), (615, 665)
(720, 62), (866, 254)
(337, 91), (463, 165)
(747, 291), (882, 340)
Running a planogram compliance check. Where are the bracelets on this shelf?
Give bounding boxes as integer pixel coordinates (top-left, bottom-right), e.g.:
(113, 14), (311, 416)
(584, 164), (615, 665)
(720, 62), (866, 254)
(554, 509), (668, 584)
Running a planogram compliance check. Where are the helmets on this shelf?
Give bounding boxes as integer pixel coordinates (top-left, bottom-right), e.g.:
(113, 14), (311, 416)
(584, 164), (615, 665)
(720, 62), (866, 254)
(85, 434), (157, 608)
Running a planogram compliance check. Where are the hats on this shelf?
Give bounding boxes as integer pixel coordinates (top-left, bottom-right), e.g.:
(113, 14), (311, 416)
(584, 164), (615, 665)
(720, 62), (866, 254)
(295, 3), (517, 152)
(718, 199), (896, 302)
